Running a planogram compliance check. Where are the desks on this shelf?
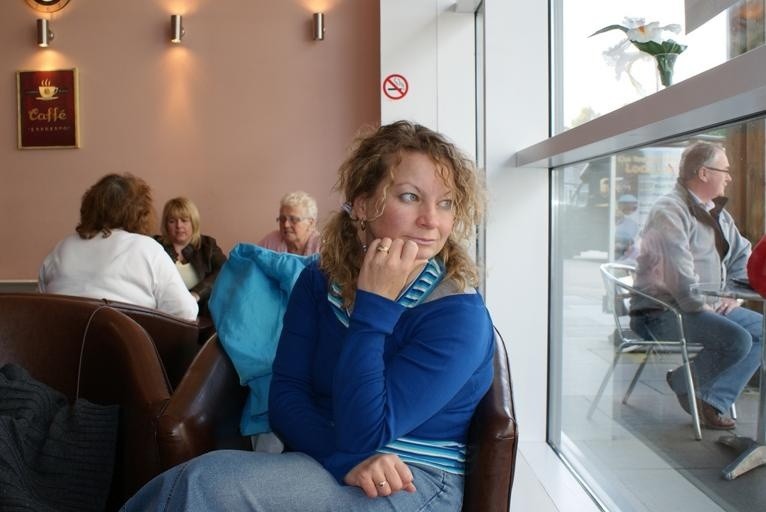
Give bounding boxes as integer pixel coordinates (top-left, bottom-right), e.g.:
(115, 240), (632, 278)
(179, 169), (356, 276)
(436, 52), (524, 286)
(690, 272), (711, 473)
(683, 275), (765, 485)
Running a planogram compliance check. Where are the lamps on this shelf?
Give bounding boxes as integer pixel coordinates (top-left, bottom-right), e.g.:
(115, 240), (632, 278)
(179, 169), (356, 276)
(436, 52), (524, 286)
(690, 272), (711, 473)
(311, 11), (325, 42)
(167, 14), (185, 44)
(34, 17), (54, 49)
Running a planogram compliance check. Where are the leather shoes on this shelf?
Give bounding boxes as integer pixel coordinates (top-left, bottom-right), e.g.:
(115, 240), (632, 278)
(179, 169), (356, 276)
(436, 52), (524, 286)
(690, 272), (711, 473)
(696, 397), (737, 430)
(666, 370), (694, 415)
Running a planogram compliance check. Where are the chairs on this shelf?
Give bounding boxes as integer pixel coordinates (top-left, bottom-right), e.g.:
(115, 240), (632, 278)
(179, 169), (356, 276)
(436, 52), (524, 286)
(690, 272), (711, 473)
(579, 259), (741, 442)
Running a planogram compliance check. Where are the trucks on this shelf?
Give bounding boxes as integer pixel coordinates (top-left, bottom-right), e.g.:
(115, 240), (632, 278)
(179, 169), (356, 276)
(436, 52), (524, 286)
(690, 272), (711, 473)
(562, 135), (727, 260)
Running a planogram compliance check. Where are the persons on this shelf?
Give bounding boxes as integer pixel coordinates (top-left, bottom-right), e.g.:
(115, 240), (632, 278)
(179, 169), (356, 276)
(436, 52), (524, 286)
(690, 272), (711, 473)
(38, 172), (198, 324)
(630, 141), (765, 430)
(114, 120), (496, 511)
(257, 190), (323, 254)
(154, 197), (227, 300)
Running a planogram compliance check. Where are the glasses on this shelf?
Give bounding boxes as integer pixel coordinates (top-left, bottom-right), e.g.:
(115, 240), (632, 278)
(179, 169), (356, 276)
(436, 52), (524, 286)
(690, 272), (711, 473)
(276, 217), (302, 223)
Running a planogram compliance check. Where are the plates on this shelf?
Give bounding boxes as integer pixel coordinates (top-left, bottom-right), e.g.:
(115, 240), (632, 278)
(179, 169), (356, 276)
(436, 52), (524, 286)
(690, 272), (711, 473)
(35, 97), (58, 101)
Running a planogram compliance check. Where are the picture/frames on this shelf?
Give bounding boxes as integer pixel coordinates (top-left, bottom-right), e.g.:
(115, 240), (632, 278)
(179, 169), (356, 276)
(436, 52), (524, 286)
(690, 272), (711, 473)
(10, 65), (80, 151)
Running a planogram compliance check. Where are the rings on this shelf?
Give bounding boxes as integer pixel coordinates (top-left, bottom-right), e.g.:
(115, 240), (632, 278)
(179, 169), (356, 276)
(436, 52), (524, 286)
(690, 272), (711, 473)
(377, 480), (387, 486)
(377, 245), (388, 251)
(724, 302), (728, 307)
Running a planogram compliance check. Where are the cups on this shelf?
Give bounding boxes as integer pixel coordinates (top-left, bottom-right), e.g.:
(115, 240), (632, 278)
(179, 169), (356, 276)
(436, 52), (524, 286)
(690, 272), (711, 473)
(38, 86), (58, 98)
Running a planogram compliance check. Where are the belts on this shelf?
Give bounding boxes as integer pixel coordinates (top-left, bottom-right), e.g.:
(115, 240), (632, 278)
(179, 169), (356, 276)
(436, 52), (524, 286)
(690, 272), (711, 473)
(629, 307), (662, 317)
